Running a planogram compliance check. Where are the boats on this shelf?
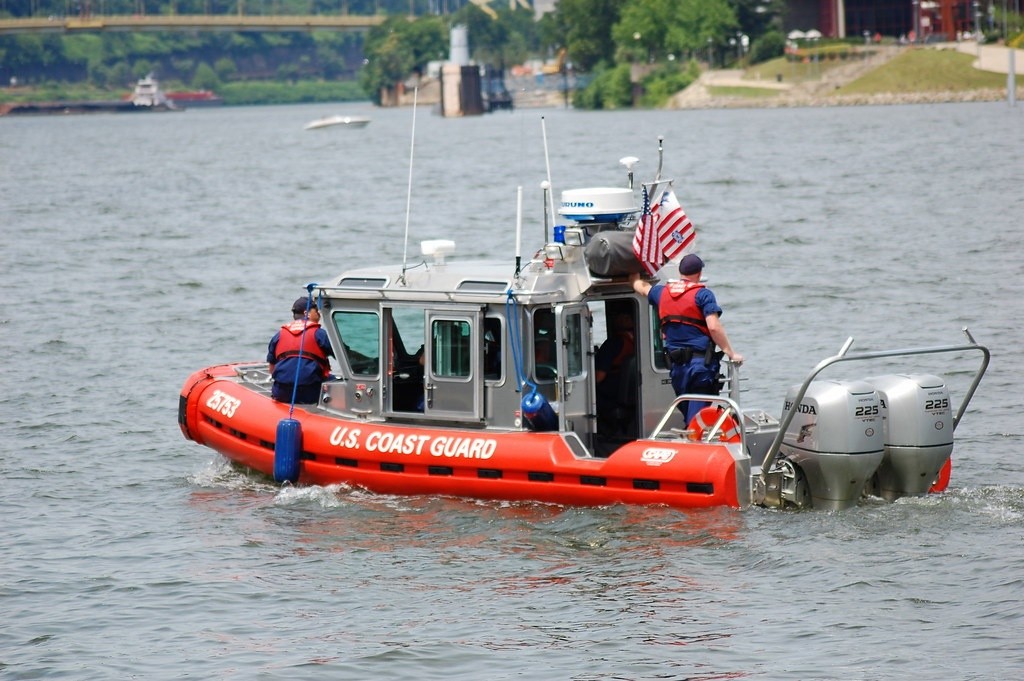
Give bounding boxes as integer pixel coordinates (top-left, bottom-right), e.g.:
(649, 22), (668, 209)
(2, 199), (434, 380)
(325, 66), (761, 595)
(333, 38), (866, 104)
(306, 117), (370, 130)
(174, 89), (993, 518)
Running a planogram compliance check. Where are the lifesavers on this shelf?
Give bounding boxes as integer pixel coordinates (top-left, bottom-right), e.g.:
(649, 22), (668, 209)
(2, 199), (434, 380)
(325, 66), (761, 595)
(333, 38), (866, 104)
(687, 406), (741, 442)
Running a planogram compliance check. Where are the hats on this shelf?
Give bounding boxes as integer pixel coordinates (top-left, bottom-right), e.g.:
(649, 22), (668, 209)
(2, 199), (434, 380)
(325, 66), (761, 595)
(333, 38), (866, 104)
(291, 296), (317, 314)
(679, 254), (705, 274)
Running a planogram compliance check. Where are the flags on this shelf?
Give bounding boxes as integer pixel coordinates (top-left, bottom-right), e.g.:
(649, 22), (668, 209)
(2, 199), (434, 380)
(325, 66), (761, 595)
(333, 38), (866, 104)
(632, 182), (697, 279)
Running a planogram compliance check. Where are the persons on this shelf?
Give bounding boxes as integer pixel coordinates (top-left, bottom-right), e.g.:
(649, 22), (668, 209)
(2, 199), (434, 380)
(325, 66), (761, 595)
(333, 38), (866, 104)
(267, 296), (350, 404)
(418, 311), (638, 442)
(630, 254), (743, 429)
